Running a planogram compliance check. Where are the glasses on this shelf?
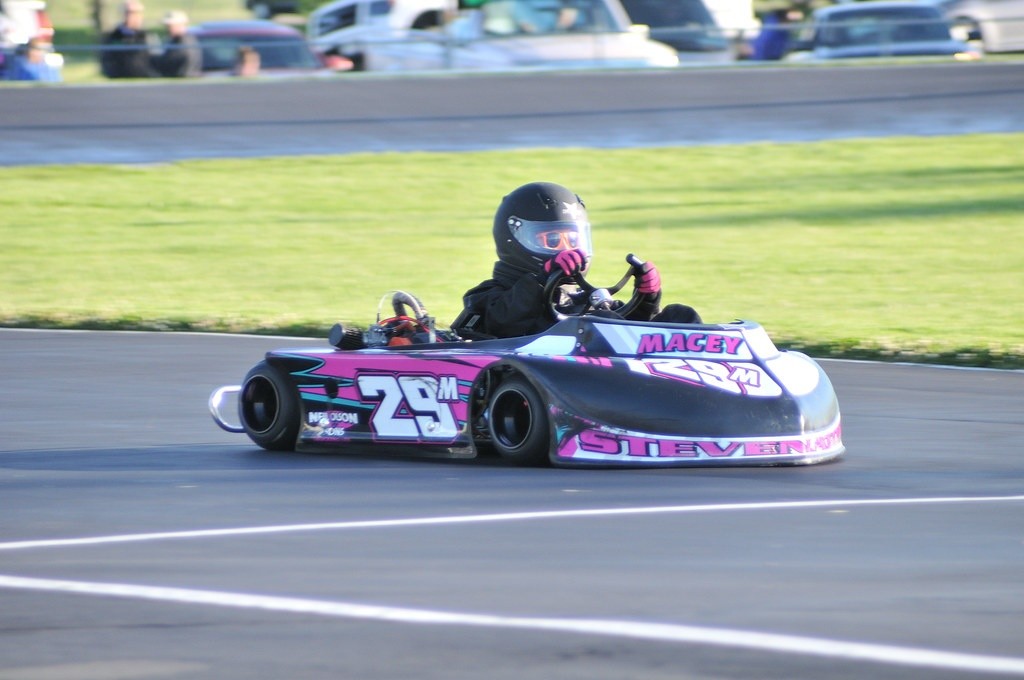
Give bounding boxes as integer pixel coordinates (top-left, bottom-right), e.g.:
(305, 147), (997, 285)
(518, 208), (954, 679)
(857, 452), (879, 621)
(535, 227), (583, 251)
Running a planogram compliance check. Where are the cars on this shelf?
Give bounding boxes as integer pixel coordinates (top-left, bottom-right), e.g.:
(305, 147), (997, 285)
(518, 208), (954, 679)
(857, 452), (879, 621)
(153, 0), (1024, 77)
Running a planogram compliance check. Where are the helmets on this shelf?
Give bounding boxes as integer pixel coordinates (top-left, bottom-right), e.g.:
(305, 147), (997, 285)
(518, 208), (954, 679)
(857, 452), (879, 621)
(492, 181), (594, 281)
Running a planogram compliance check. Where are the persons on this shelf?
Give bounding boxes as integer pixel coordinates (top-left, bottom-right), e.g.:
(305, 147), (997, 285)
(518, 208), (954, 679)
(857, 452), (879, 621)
(480, 182), (704, 340)
(0, 1), (812, 81)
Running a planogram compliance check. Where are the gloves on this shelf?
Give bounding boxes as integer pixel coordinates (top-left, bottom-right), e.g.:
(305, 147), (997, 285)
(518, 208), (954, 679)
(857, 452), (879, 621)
(545, 246), (588, 275)
(634, 260), (663, 299)
